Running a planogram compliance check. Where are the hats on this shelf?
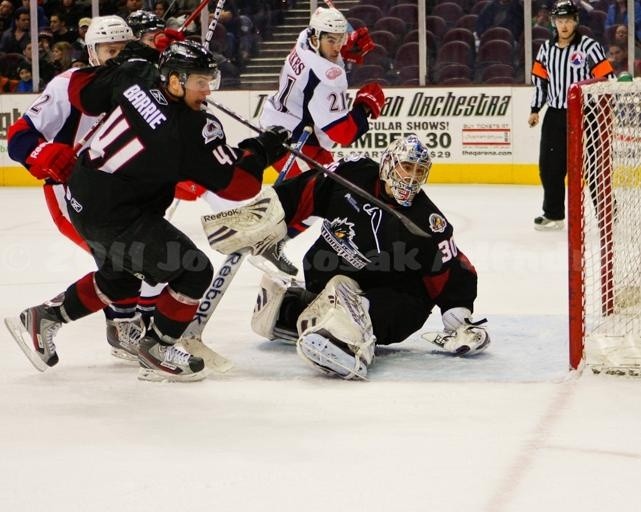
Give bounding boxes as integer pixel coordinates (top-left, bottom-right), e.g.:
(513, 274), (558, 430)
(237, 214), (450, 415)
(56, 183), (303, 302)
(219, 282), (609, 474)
(79, 18), (90, 28)
(15, 58), (32, 74)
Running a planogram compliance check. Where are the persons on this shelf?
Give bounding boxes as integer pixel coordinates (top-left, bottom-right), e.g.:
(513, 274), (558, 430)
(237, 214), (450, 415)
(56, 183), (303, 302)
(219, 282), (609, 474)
(529, 3), (618, 229)
(2, 15), (291, 384)
(1, 0), (286, 91)
(244, 6), (387, 278)
(203, 137), (491, 379)
(531, 0), (641, 81)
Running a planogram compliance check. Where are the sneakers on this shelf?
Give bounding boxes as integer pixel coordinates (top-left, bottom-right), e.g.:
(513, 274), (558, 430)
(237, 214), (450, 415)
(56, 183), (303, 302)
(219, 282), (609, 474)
(534, 216), (552, 225)
(20, 292), (67, 367)
(104, 311), (145, 355)
(137, 318), (203, 374)
(301, 334), (367, 380)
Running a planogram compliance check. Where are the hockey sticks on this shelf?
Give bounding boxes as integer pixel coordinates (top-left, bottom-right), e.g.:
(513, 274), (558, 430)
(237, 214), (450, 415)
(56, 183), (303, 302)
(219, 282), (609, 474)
(180, 126), (312, 372)
(204, 95), (430, 237)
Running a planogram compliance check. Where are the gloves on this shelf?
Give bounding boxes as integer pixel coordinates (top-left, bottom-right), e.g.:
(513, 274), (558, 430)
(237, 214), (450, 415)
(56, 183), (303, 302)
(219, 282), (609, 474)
(155, 29), (184, 53)
(353, 82), (387, 117)
(342, 29), (375, 67)
(239, 127), (288, 167)
(26, 142), (76, 183)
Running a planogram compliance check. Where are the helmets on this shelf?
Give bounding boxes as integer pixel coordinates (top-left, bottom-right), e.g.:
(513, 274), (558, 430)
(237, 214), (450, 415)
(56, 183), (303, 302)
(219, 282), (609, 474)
(308, 7), (346, 42)
(553, 3), (579, 21)
(157, 40), (216, 94)
(379, 135), (432, 207)
(128, 9), (162, 40)
(84, 15), (137, 67)
